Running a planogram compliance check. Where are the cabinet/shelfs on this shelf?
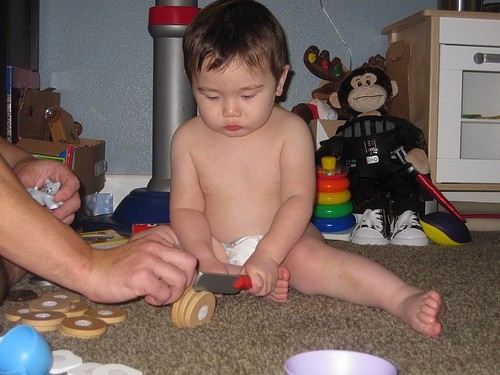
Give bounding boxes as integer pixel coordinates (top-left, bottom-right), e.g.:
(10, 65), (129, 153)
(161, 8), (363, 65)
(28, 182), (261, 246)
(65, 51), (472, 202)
(382, 9), (500, 231)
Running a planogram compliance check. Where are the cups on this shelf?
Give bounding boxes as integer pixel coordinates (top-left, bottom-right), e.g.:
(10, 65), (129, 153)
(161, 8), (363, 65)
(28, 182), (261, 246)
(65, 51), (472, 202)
(0, 324), (53, 375)
(284, 349), (397, 375)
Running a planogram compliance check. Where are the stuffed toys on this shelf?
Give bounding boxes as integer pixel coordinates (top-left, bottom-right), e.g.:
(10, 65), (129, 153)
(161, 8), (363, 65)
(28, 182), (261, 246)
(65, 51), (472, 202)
(315, 62), (436, 245)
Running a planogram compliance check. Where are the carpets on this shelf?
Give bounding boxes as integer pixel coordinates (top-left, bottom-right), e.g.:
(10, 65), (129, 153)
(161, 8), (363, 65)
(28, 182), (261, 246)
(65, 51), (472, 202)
(0, 230), (500, 375)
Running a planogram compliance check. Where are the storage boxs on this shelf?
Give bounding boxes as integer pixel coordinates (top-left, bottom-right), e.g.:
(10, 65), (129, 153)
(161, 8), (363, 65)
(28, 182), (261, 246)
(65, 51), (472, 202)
(15, 85), (108, 196)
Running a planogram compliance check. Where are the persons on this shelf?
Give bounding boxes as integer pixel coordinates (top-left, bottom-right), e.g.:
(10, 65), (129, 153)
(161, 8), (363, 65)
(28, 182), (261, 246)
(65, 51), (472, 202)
(168, 0), (443, 337)
(0, 137), (198, 308)
